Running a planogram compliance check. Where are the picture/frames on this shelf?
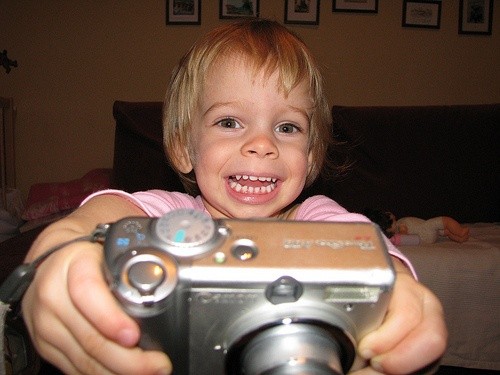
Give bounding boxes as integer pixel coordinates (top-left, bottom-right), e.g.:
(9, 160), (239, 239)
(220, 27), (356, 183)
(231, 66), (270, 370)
(219, 0), (260, 20)
(332, 0), (379, 15)
(458, 0), (494, 36)
(165, 0), (201, 26)
(284, 0), (321, 26)
(402, 0), (442, 30)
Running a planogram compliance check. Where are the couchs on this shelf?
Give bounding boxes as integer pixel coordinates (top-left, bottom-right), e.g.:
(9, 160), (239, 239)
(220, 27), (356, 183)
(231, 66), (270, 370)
(0, 98), (500, 375)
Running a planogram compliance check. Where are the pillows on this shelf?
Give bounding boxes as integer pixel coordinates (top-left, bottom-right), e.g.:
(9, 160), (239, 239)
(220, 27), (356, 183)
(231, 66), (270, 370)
(21, 168), (116, 221)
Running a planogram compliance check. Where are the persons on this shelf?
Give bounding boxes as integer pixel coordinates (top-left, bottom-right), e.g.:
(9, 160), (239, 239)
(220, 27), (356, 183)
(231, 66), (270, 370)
(22, 19), (449, 375)
(358, 207), (470, 244)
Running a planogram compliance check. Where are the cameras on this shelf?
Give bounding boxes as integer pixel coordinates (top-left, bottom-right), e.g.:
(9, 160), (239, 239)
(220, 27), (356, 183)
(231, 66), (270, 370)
(103, 208), (395, 375)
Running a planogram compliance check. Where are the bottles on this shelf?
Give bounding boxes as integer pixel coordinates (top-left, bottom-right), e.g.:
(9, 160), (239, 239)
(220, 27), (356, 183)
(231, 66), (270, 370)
(389, 233), (420, 246)
